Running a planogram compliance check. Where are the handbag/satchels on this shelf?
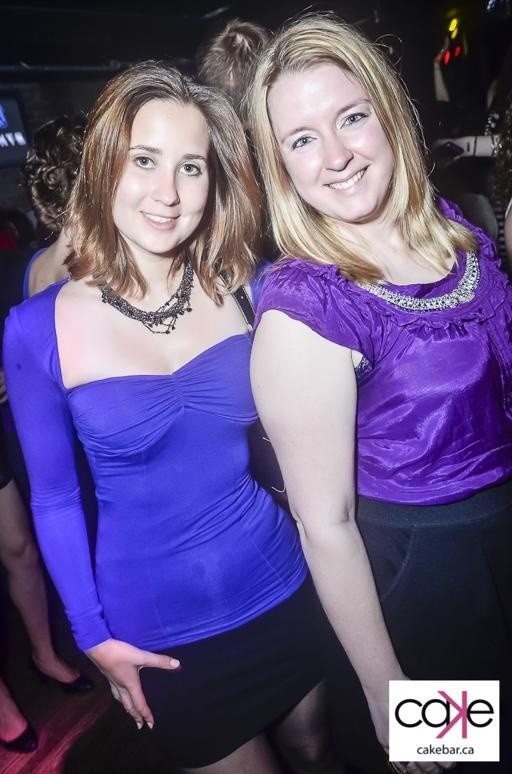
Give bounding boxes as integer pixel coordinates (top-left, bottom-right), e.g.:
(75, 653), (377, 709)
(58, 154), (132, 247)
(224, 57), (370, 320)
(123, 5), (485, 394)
(246, 419), (291, 516)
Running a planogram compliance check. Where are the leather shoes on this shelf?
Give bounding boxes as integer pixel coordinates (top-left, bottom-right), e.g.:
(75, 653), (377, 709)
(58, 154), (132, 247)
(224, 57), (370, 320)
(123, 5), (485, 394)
(0, 721), (38, 752)
(26, 656), (94, 693)
